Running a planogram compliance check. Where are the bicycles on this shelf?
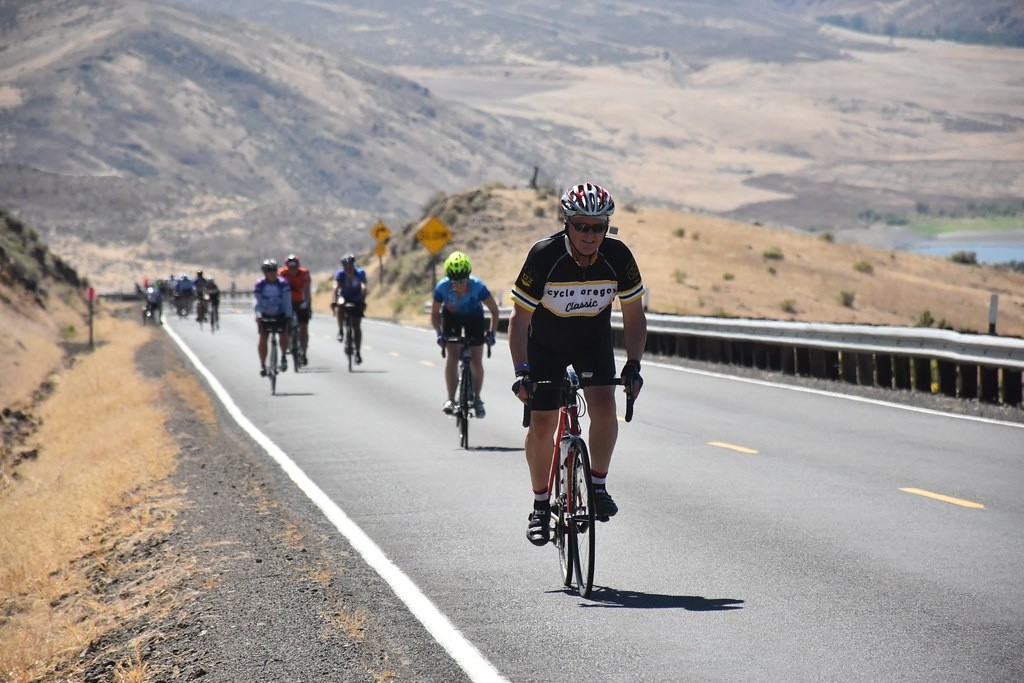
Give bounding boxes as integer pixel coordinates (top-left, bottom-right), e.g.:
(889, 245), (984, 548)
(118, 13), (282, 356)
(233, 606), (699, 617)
(284, 301), (312, 372)
(438, 335), (491, 449)
(331, 301), (366, 372)
(517, 374), (635, 598)
(257, 317), (292, 395)
(140, 290), (219, 332)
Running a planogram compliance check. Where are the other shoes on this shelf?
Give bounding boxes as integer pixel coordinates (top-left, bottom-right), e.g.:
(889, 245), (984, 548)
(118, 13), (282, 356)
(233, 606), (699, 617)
(300, 351), (307, 365)
(281, 360), (287, 372)
(472, 400), (485, 418)
(260, 369), (267, 377)
(338, 331), (344, 341)
(442, 400), (455, 413)
(355, 351), (361, 364)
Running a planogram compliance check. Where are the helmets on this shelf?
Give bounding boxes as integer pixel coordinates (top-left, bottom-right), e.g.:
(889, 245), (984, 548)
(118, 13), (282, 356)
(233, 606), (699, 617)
(445, 251), (473, 282)
(560, 182), (615, 222)
(206, 277), (213, 284)
(261, 260), (278, 272)
(284, 255), (300, 268)
(198, 271), (203, 277)
(341, 253), (354, 265)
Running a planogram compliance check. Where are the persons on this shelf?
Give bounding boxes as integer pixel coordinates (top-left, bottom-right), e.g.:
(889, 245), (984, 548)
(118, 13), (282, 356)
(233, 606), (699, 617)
(253, 259), (292, 376)
(508, 183), (647, 546)
(149, 269), (221, 323)
(431, 251), (500, 418)
(331, 253), (369, 362)
(278, 255), (311, 366)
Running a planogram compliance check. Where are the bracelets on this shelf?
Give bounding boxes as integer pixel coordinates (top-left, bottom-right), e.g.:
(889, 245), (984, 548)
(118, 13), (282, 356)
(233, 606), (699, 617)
(515, 363), (529, 370)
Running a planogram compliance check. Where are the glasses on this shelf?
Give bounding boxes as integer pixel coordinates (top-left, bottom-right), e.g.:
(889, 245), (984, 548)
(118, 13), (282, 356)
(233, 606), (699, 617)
(568, 216), (609, 233)
(451, 279), (468, 285)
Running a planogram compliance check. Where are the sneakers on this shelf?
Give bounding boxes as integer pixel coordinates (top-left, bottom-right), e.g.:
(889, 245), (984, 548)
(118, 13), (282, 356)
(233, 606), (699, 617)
(594, 490), (618, 517)
(526, 508), (551, 547)
